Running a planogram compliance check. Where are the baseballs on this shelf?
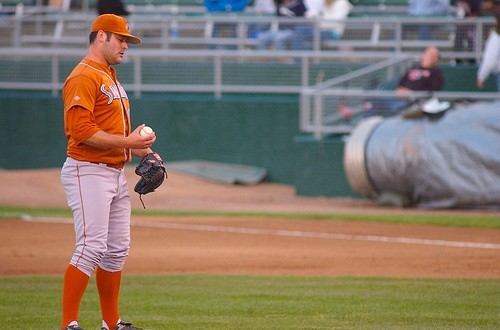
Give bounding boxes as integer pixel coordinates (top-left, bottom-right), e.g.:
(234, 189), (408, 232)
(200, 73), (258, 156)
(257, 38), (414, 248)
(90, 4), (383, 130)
(139, 126), (153, 136)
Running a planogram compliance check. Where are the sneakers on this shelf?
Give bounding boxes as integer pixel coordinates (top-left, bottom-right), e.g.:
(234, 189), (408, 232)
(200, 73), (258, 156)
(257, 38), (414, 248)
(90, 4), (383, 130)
(101, 316), (144, 330)
(65, 320), (82, 330)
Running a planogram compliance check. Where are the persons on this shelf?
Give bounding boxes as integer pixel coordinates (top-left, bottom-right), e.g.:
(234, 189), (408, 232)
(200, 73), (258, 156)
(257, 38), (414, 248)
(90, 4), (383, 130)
(254, 0), (500, 123)
(62, 14), (164, 330)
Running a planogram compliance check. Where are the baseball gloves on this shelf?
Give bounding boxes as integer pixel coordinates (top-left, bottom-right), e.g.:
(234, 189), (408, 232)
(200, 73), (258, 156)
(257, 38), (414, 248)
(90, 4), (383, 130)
(133, 152), (167, 194)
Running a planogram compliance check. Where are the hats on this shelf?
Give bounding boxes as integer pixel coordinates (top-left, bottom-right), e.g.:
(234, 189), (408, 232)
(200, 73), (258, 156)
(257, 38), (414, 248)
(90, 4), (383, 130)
(90, 13), (142, 45)
